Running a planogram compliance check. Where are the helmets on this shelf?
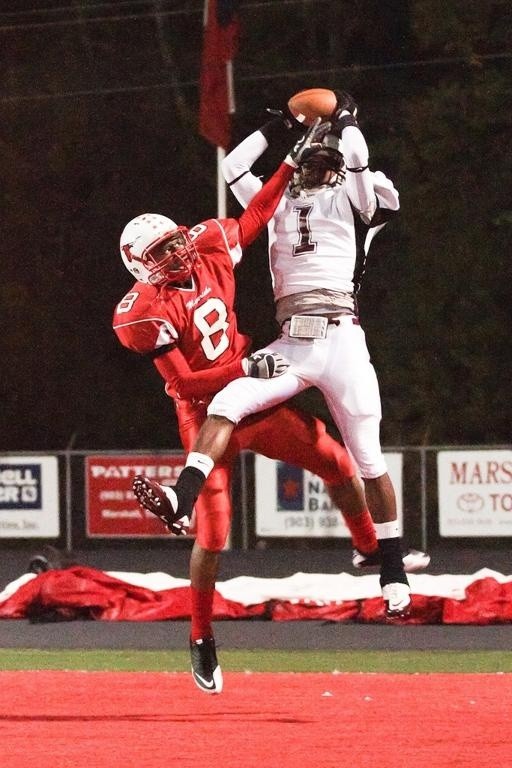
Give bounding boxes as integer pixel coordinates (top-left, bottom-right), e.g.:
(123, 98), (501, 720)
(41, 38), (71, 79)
(287, 133), (346, 198)
(119, 212), (198, 287)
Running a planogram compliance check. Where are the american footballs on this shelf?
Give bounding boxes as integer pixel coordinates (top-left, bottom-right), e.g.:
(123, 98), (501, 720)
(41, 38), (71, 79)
(286, 87), (339, 125)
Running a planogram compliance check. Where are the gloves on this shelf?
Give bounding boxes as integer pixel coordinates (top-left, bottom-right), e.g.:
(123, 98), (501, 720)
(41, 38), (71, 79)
(288, 117), (332, 170)
(246, 350), (291, 379)
(332, 89), (359, 135)
(268, 105), (306, 131)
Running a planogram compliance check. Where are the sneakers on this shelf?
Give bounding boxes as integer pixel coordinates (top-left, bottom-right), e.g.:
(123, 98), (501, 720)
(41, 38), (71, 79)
(352, 546), (431, 572)
(189, 636), (224, 695)
(131, 474), (191, 537)
(379, 568), (414, 623)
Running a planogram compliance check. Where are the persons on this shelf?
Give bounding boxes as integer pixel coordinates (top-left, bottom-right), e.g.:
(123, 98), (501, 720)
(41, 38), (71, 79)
(113, 117), (433, 696)
(132, 89), (414, 622)
